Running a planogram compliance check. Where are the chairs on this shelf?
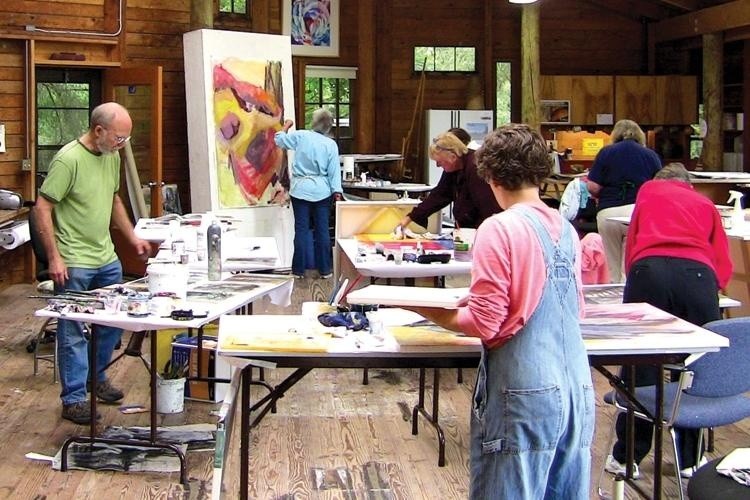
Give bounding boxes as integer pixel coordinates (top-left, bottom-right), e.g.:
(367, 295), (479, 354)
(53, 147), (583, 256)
(595, 318), (750, 499)
(686, 450), (749, 500)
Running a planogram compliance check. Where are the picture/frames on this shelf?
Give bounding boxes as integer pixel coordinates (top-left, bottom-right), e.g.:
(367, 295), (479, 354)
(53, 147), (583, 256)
(283, 1), (340, 58)
(539, 99), (571, 125)
(335, 201), (442, 305)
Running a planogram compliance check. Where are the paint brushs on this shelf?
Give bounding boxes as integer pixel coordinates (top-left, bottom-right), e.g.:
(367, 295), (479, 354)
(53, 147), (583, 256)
(158, 311), (209, 319)
(54, 292), (97, 298)
(216, 214), (243, 233)
(152, 217), (183, 223)
(27, 296), (76, 302)
(146, 222), (171, 225)
(328, 270), (361, 306)
(140, 227), (174, 229)
(230, 267), (293, 276)
(99, 286), (118, 291)
(138, 353), (190, 380)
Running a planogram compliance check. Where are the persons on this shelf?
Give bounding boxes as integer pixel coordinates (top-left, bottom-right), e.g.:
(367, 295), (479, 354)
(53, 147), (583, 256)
(603, 159), (733, 479)
(377, 123), (595, 500)
(32, 101), (152, 424)
(272, 107), (342, 280)
(392, 133), (503, 237)
(588, 117), (662, 284)
(448, 125), (485, 151)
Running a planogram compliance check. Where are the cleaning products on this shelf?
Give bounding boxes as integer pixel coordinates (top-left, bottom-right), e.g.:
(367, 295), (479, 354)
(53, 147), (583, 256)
(725, 191), (745, 230)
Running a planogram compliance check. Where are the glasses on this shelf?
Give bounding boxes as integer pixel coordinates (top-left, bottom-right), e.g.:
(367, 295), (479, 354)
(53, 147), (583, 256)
(103, 127), (132, 143)
(432, 137), (455, 152)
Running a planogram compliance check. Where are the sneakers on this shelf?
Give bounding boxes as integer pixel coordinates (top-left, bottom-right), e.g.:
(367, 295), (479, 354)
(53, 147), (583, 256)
(319, 272), (333, 279)
(680, 456), (709, 478)
(289, 271), (304, 279)
(62, 402), (101, 424)
(604, 454), (640, 479)
(86, 381), (123, 402)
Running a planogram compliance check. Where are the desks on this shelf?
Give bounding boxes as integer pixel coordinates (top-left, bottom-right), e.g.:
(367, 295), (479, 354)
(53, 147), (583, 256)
(217, 309), (729, 499)
(336, 237), (473, 286)
(34, 273), (294, 483)
(347, 284), (742, 466)
